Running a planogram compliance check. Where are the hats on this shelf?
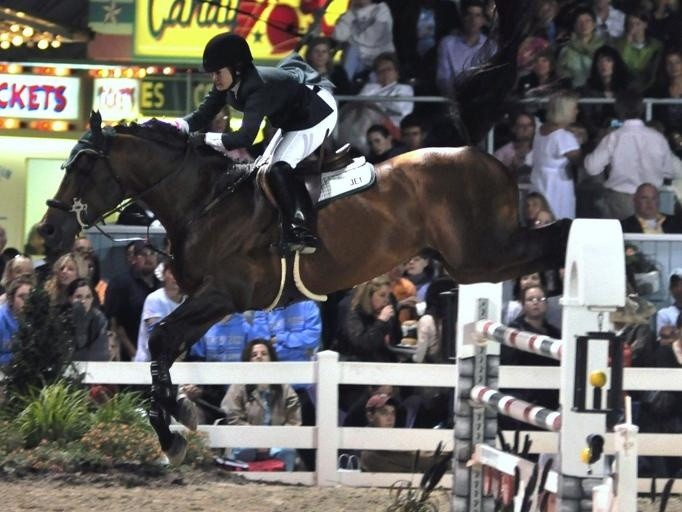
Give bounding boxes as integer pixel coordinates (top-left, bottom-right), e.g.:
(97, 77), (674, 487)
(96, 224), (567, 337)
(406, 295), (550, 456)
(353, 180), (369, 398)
(366, 392), (400, 410)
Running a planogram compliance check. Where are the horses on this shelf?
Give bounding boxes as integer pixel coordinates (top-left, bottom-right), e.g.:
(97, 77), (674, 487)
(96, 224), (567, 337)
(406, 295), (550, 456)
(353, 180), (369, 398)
(42, 0), (660, 459)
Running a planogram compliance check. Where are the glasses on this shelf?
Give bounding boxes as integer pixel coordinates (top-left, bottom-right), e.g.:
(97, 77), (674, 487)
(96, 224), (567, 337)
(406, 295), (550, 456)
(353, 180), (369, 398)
(524, 295), (547, 302)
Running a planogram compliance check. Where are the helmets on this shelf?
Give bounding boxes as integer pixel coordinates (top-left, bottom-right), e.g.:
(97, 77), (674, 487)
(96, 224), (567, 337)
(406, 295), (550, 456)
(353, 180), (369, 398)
(202, 33), (253, 73)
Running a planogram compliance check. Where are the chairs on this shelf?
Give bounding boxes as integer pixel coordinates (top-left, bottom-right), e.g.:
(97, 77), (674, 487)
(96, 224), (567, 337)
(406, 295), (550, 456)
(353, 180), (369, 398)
(187, 246), (680, 473)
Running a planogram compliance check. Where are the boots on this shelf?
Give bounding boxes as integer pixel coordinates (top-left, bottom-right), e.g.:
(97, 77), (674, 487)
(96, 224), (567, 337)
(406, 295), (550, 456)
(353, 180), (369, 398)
(265, 160), (320, 254)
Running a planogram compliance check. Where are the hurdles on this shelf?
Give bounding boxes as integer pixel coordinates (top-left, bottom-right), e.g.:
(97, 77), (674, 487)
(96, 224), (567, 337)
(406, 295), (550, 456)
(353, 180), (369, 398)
(451, 218), (639, 512)
